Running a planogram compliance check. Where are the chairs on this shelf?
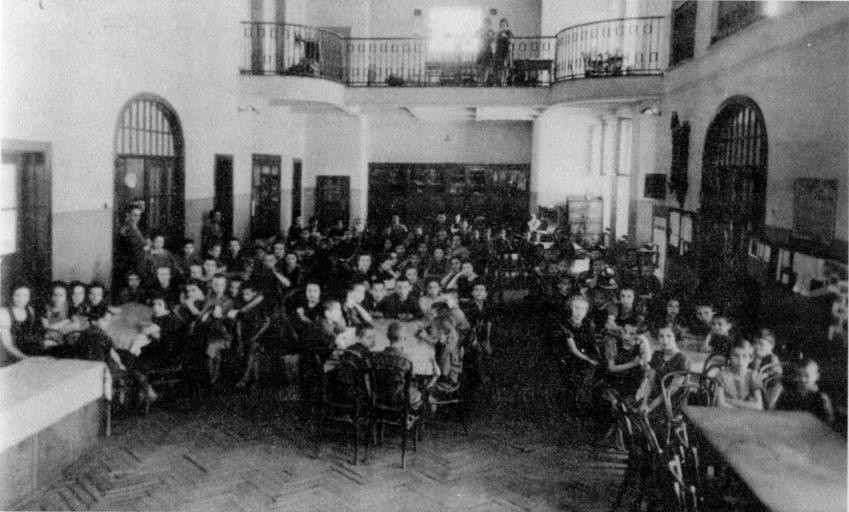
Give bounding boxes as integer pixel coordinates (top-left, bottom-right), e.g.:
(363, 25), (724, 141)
(609, 390), (669, 506)
(663, 436), (716, 511)
(34, 328), (66, 354)
(314, 347), (375, 464)
(760, 361), (795, 394)
(64, 331), (152, 433)
(662, 370), (717, 467)
(700, 351), (736, 391)
(360, 354), (421, 468)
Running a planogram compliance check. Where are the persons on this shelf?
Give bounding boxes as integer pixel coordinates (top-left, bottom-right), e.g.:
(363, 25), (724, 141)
(529, 212), (832, 449)
(0, 204), (533, 445)
(475, 19), (496, 86)
(495, 19), (513, 85)
(294, 37), (320, 73)
(583, 47), (624, 77)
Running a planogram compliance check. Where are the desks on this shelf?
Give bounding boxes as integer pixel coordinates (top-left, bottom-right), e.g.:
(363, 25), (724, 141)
(0, 355), (111, 510)
(513, 59), (553, 86)
(681, 408), (848, 511)
(42, 305), (160, 363)
(334, 320), (442, 402)
